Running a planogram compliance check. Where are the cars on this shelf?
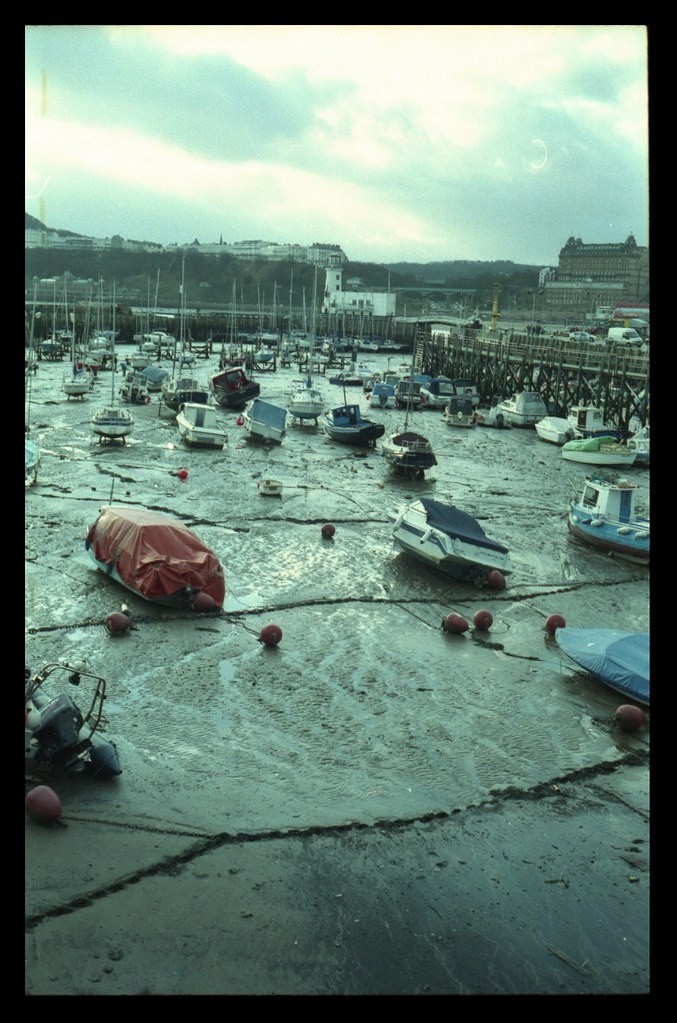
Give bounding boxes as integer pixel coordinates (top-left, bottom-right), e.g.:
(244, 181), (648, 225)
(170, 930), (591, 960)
(570, 332), (597, 342)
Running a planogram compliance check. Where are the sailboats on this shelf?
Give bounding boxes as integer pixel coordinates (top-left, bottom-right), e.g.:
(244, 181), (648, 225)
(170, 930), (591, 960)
(25, 256), (650, 785)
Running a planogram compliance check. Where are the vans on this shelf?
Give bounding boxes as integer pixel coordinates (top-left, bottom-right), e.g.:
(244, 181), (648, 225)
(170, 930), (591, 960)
(608, 328), (643, 348)
